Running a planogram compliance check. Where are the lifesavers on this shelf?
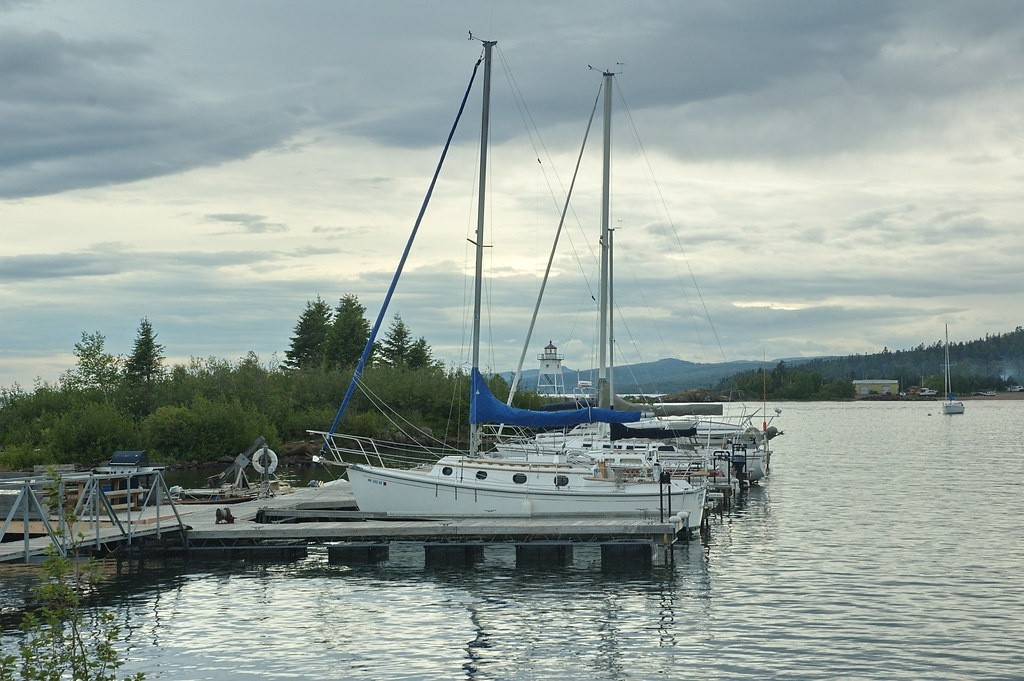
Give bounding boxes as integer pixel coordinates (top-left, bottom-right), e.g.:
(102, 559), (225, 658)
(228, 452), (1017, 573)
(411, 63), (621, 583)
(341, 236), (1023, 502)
(251, 447), (279, 476)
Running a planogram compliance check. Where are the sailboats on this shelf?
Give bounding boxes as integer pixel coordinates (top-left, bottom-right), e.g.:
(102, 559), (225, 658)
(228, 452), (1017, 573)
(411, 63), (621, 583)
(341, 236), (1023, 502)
(941, 323), (965, 414)
(305, 28), (788, 537)
